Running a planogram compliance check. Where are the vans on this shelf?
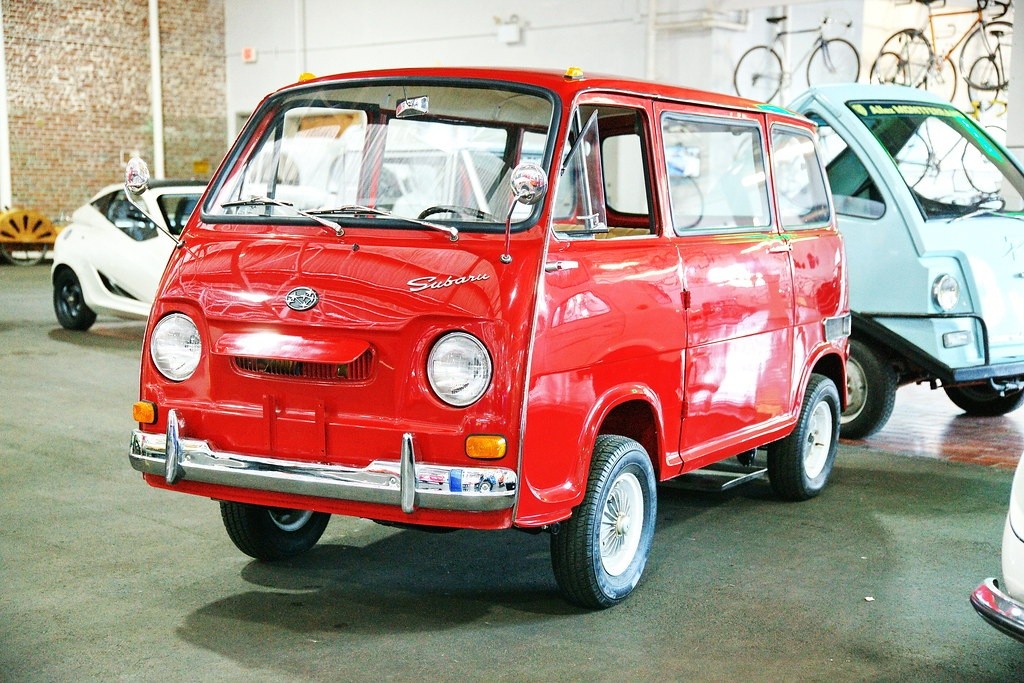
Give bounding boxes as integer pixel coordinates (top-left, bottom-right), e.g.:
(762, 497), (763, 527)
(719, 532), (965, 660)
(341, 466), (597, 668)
(125, 60), (850, 610)
(311, 84), (1023, 442)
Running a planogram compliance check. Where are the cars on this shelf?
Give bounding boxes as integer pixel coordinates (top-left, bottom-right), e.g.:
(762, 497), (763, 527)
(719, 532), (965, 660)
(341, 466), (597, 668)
(51, 178), (337, 333)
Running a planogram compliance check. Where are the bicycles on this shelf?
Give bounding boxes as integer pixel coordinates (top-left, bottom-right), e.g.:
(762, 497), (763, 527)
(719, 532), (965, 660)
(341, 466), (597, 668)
(734, 8), (860, 104)
(869, 1), (1013, 195)
